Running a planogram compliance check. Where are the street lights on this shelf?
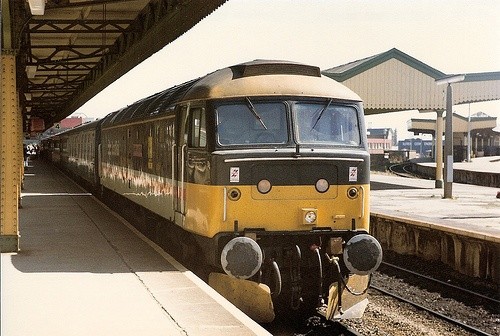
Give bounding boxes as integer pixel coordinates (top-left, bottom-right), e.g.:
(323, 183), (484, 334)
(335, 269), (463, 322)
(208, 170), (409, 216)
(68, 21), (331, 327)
(435, 73), (465, 198)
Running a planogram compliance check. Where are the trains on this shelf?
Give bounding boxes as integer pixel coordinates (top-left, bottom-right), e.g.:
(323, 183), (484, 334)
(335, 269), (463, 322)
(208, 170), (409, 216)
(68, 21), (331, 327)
(37, 59), (373, 322)
